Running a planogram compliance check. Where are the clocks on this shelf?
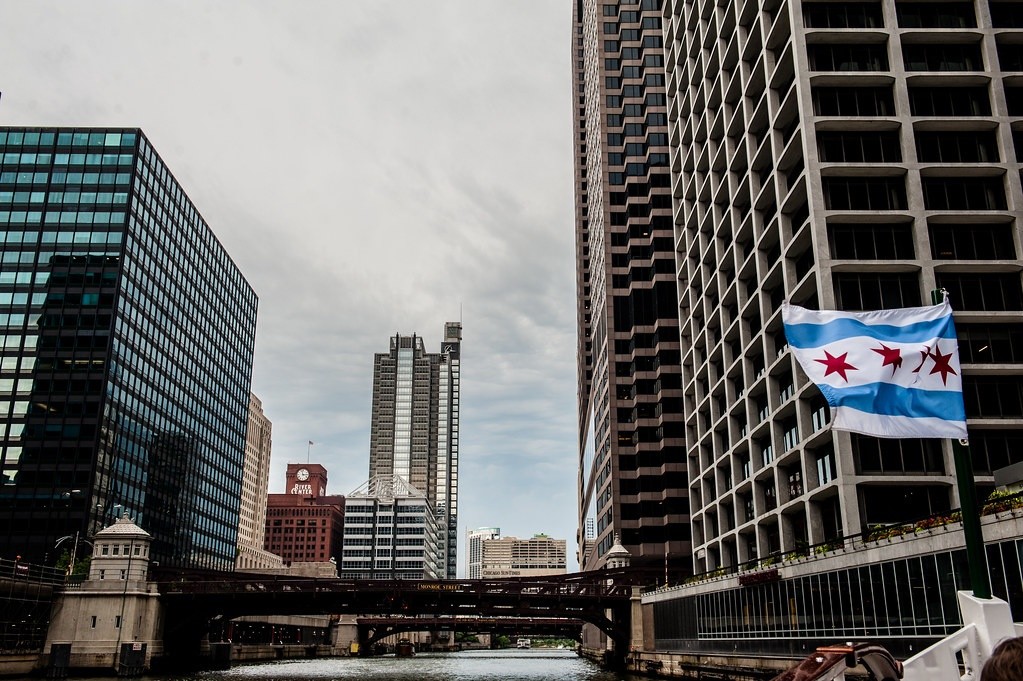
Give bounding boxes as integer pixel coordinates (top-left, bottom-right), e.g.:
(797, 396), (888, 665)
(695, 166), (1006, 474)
(297, 468), (310, 481)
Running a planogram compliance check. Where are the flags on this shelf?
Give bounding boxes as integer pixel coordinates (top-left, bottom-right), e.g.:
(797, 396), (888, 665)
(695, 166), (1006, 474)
(782, 301), (969, 441)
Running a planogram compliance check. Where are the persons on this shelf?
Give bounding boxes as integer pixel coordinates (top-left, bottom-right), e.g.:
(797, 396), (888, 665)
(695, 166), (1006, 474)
(980, 636), (1023, 681)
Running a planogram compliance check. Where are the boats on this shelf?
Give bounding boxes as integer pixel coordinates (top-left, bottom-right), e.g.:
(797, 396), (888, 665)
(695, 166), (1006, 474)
(517, 639), (531, 649)
(395, 637), (416, 658)
